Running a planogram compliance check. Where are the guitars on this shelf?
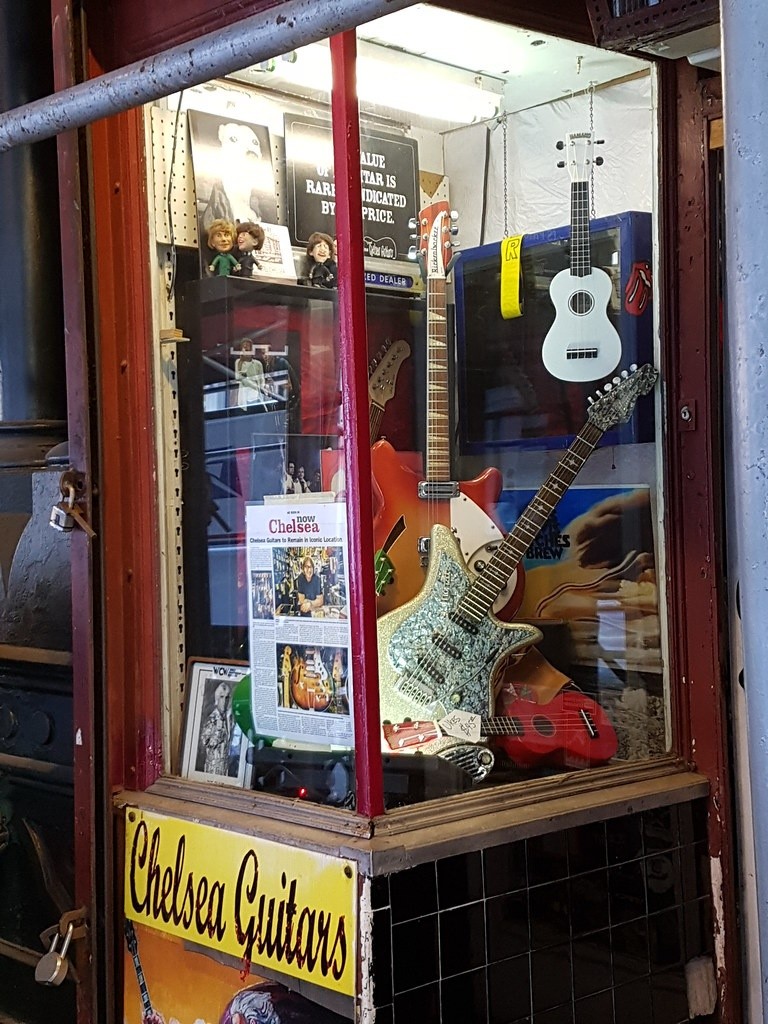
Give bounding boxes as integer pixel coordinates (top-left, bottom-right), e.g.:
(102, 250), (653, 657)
(370, 174), (526, 620)
(383, 690), (619, 769)
(228, 332), (417, 748)
(540, 129), (622, 382)
(365, 365), (661, 759)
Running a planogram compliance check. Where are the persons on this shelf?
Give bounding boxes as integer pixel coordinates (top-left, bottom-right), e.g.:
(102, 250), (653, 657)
(306, 231), (339, 290)
(207, 218), (265, 278)
(234, 336), (301, 412)
(297, 556), (325, 616)
(199, 682), (235, 777)
